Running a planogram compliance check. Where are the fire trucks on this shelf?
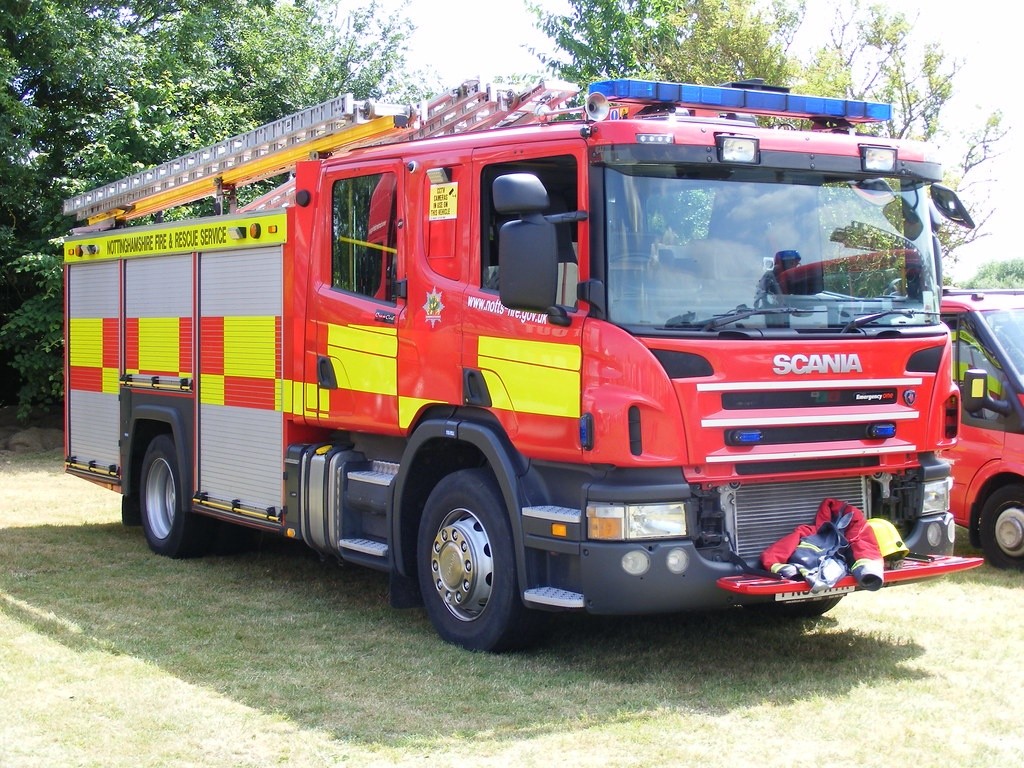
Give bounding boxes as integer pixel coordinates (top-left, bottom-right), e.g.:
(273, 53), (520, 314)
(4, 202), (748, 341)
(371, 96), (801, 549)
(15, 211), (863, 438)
(60, 78), (982, 653)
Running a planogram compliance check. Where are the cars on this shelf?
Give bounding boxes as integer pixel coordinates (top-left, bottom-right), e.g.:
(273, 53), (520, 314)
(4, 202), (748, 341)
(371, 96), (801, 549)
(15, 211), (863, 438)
(938, 291), (1024, 571)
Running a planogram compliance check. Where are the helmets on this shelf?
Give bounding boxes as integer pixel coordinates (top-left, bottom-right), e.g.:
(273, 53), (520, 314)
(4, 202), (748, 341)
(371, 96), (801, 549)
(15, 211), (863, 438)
(866, 518), (909, 568)
